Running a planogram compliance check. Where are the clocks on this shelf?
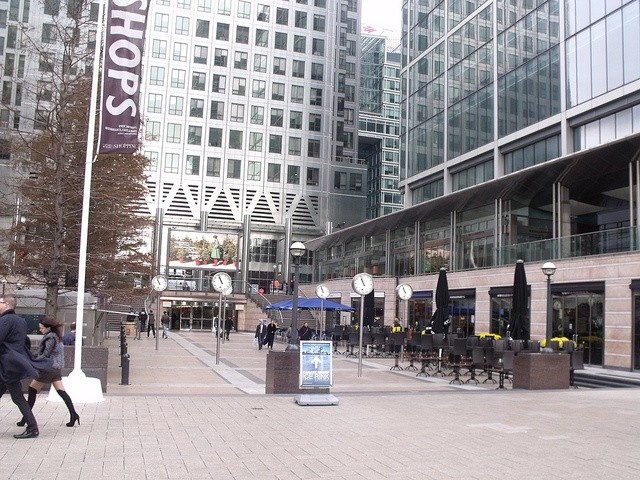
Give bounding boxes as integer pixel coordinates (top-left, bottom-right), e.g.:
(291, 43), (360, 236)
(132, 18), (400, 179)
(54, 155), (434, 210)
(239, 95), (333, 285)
(213, 272), (231, 291)
(151, 275), (166, 291)
(552, 299), (563, 310)
(222, 284), (233, 296)
(314, 284), (328, 298)
(352, 272), (374, 296)
(396, 285), (412, 300)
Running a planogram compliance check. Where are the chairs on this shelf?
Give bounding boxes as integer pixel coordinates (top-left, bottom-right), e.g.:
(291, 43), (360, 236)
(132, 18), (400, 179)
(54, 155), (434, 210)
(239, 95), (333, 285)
(331, 325), (585, 389)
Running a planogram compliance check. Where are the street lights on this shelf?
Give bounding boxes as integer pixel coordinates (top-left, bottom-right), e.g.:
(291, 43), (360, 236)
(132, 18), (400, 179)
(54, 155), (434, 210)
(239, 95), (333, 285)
(273, 265), (276, 279)
(286, 241), (306, 351)
(541, 262), (556, 352)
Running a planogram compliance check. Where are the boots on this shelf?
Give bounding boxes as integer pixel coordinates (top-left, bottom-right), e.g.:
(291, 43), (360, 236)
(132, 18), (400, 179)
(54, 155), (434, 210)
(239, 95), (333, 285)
(17, 386), (37, 427)
(57, 389), (80, 427)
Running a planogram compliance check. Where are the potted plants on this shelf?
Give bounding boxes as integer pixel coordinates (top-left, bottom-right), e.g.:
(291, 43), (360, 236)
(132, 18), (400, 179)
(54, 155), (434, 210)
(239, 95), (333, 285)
(222, 254), (229, 265)
(195, 257), (201, 266)
(233, 256), (238, 267)
(169, 247), (177, 261)
(177, 247), (188, 262)
(200, 250), (210, 264)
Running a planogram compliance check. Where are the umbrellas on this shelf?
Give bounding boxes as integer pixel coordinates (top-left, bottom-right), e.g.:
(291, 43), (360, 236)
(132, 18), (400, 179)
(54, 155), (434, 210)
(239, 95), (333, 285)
(279, 297), (310, 340)
(363, 288), (374, 356)
(264, 297), (292, 337)
(509, 259), (528, 356)
(294, 297), (357, 340)
(430, 268), (449, 372)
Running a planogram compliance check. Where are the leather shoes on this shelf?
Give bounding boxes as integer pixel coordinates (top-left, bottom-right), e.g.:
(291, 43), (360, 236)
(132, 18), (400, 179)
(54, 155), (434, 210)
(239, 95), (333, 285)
(13, 430), (39, 439)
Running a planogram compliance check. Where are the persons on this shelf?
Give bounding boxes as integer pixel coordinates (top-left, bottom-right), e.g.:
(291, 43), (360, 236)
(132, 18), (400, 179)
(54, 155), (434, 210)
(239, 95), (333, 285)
(147, 309), (156, 338)
(413, 320), (419, 333)
(521, 326), (529, 349)
(0, 294), (40, 439)
(225, 315), (234, 340)
(161, 311), (170, 338)
(298, 322), (313, 340)
(62, 321), (83, 347)
(17, 316), (80, 427)
(265, 319), (276, 349)
(274, 279), (279, 290)
(354, 323), (358, 332)
(406, 326), (414, 352)
(259, 288), (264, 295)
(255, 319), (266, 350)
(139, 308), (146, 332)
(211, 235), (221, 266)
(127, 307), (135, 322)
(214, 314), (223, 338)
(463, 318), (473, 339)
(388, 318), (402, 357)
(287, 323), (293, 344)
